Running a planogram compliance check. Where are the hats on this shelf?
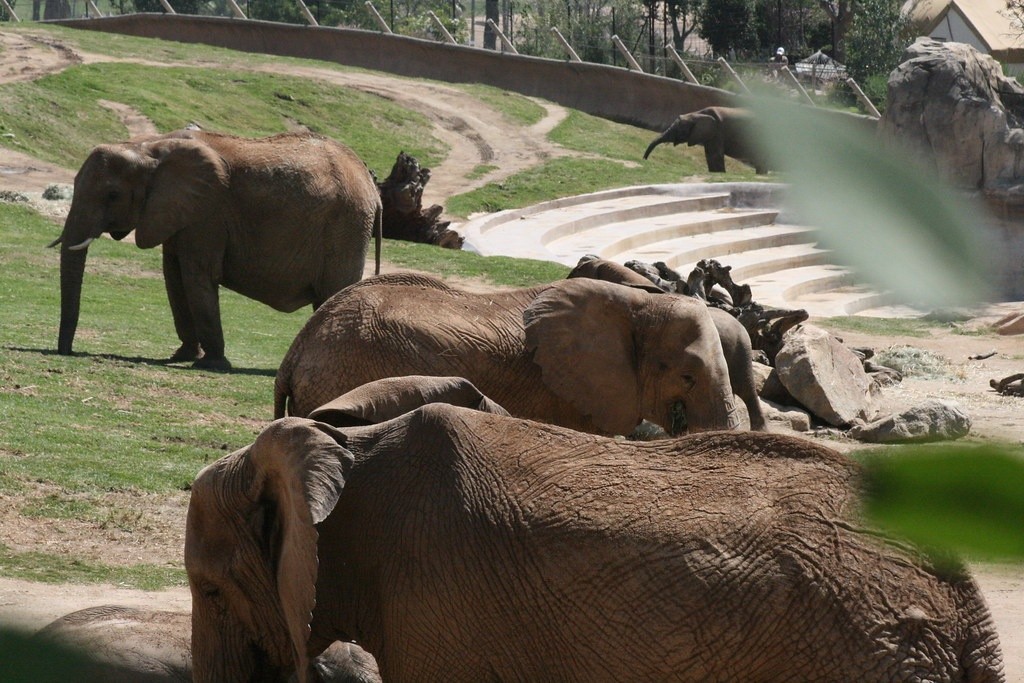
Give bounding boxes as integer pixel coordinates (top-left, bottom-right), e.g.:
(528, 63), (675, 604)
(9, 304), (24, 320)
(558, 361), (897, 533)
(776, 47), (784, 55)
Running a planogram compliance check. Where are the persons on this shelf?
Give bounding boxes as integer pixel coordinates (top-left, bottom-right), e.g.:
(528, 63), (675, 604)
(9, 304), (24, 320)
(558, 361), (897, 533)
(769, 47), (788, 77)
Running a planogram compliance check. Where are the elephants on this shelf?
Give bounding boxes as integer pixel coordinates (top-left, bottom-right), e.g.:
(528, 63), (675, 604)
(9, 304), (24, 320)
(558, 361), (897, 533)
(182, 253), (1008, 683)
(642, 108), (786, 175)
(47, 127), (385, 376)
